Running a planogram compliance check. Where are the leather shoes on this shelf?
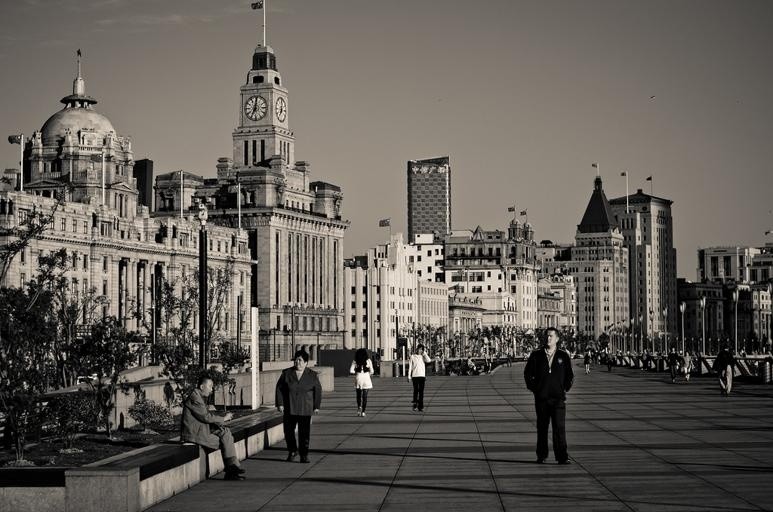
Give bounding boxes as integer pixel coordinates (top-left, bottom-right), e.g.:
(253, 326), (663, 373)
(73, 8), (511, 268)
(223, 464), (246, 480)
(557, 459), (572, 464)
(535, 458), (546, 464)
(300, 455), (310, 464)
(286, 451), (297, 462)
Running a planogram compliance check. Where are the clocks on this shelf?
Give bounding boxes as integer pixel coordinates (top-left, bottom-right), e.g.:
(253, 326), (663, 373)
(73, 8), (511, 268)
(276, 96), (289, 123)
(243, 95), (266, 121)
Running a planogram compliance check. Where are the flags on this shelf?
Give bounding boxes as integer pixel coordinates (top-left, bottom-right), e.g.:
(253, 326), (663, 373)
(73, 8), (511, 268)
(646, 177), (652, 181)
(520, 211), (526, 216)
(251, 1), (263, 10)
(8, 134), (20, 145)
(378, 218), (390, 227)
(621, 171), (626, 176)
(227, 184), (238, 195)
(592, 163), (597, 167)
(508, 207), (514, 212)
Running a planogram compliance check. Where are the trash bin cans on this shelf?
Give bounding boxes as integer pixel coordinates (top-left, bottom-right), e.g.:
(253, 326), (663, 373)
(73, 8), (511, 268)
(393, 362), (400, 378)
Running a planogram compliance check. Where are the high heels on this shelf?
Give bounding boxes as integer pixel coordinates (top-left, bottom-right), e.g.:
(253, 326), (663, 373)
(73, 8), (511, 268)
(357, 409), (367, 418)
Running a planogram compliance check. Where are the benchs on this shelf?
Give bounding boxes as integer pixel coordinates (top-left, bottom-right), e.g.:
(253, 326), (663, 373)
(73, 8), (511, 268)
(62, 407), (299, 511)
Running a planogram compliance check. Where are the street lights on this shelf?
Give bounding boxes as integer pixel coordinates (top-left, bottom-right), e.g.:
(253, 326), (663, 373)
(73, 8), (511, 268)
(733, 287), (739, 355)
(767, 277), (773, 354)
(608, 296), (705, 356)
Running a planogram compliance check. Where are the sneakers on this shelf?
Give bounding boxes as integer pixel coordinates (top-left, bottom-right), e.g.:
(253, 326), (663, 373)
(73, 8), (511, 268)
(413, 405), (424, 412)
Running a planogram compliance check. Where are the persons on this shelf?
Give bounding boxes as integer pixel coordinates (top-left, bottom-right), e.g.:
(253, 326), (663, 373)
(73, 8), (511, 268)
(349, 348), (374, 417)
(406, 344), (431, 411)
(681, 351), (693, 382)
(467, 357), (477, 375)
(583, 354), (590, 375)
(274, 350), (321, 463)
(666, 346), (680, 383)
(641, 348), (649, 372)
(713, 348), (734, 397)
(523, 326), (573, 465)
(179, 376), (245, 481)
